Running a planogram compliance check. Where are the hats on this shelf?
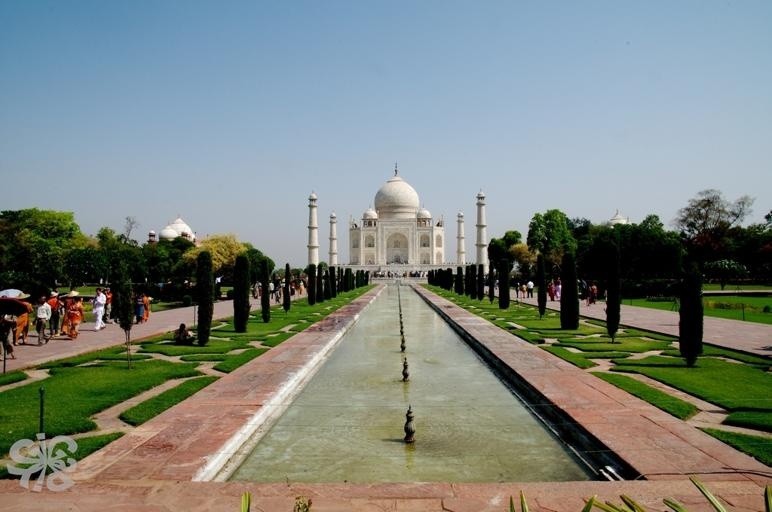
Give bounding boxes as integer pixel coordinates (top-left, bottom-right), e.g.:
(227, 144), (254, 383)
(68, 290), (79, 297)
(50, 290), (58, 298)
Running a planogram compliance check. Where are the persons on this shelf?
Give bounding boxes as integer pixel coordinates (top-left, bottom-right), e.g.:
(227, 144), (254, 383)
(495, 279), (499, 289)
(253, 278), (308, 304)
(515, 278), (607, 312)
(370, 269), (426, 278)
(0, 285), (149, 360)
(174, 324), (195, 345)
